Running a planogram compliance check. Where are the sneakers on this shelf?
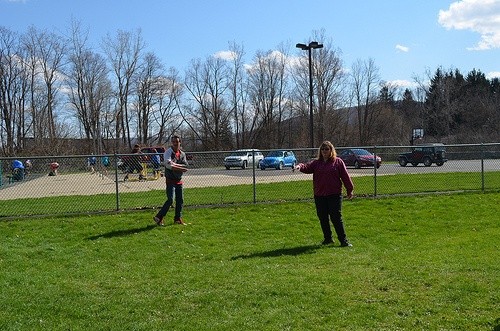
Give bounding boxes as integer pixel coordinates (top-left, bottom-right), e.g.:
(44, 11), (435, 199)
(174, 219), (185, 224)
(341, 242), (353, 247)
(322, 238), (333, 245)
(153, 217), (163, 225)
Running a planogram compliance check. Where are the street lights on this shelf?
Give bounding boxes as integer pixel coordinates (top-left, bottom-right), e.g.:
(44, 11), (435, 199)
(296, 42), (324, 149)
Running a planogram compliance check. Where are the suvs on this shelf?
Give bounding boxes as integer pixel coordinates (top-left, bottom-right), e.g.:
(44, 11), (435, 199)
(118, 146), (167, 170)
(224, 149), (264, 170)
(399, 142), (445, 167)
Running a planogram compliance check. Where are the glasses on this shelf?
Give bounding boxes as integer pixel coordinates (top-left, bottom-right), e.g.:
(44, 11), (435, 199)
(320, 148), (332, 150)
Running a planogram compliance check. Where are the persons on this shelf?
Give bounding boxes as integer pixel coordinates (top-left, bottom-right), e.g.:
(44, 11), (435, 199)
(88, 152), (97, 174)
(101, 151), (109, 176)
(153, 136), (190, 226)
(152, 148), (163, 180)
(294, 141), (354, 247)
(124, 144), (147, 181)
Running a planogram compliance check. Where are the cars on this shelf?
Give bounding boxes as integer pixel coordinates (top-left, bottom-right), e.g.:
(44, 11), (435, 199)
(257, 151), (297, 170)
(341, 149), (380, 168)
